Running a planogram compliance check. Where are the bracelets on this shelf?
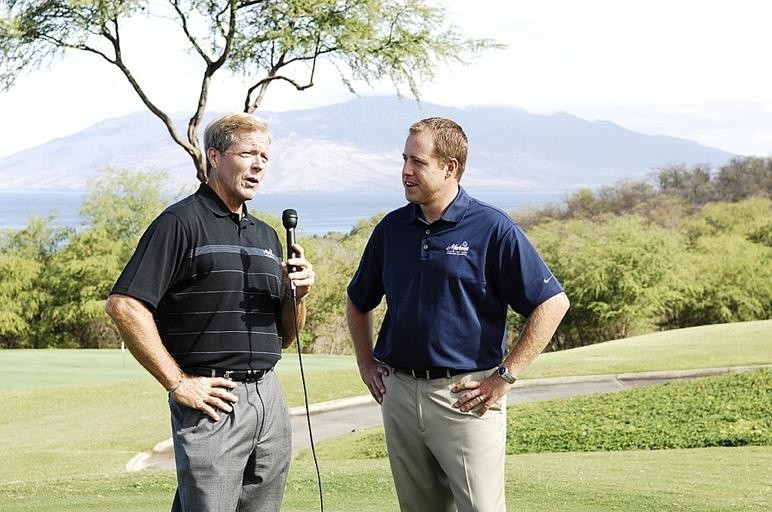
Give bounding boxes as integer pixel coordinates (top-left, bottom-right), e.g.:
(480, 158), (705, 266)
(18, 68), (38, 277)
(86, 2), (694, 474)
(165, 371), (186, 393)
(285, 291), (309, 306)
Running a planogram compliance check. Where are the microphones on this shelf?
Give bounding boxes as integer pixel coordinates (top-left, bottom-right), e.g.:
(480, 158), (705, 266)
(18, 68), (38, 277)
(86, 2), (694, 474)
(282, 208), (300, 301)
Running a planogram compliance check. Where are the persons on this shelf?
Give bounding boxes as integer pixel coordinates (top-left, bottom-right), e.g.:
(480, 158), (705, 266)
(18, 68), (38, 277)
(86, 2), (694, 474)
(104, 111), (316, 512)
(345, 114), (571, 512)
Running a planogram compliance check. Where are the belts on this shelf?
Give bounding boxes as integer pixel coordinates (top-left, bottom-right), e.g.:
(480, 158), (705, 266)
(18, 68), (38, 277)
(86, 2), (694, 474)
(391, 367), (472, 380)
(183, 366), (273, 383)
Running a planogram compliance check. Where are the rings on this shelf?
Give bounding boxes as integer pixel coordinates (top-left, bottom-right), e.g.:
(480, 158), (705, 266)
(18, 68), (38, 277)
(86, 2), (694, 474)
(478, 394), (485, 402)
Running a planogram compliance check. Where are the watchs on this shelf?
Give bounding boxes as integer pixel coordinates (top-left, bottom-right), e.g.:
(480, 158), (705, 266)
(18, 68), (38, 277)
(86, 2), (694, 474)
(496, 363), (517, 384)
